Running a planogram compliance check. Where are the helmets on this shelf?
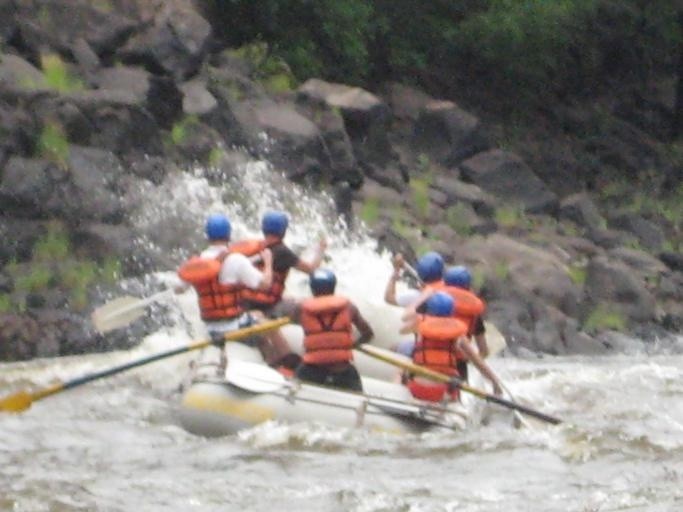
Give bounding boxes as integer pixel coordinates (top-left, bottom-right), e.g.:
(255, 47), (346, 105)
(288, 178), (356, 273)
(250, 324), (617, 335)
(426, 293), (453, 315)
(444, 267), (470, 287)
(207, 216), (230, 237)
(262, 213), (287, 237)
(310, 269), (335, 294)
(416, 253), (442, 280)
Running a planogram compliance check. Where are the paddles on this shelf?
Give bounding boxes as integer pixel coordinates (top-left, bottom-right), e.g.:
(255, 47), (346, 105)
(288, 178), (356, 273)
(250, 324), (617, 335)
(91, 252), (263, 332)
(1, 316), (291, 413)
(459, 336), (535, 433)
(223, 360), (467, 420)
(402, 265), (505, 357)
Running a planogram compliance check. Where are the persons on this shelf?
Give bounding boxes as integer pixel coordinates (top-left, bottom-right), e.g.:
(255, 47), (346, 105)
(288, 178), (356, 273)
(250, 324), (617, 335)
(384, 249), (503, 403)
(177, 209), (376, 395)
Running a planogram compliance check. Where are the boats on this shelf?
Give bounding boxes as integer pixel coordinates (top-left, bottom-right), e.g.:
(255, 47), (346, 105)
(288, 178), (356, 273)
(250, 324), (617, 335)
(183, 279), (493, 460)
